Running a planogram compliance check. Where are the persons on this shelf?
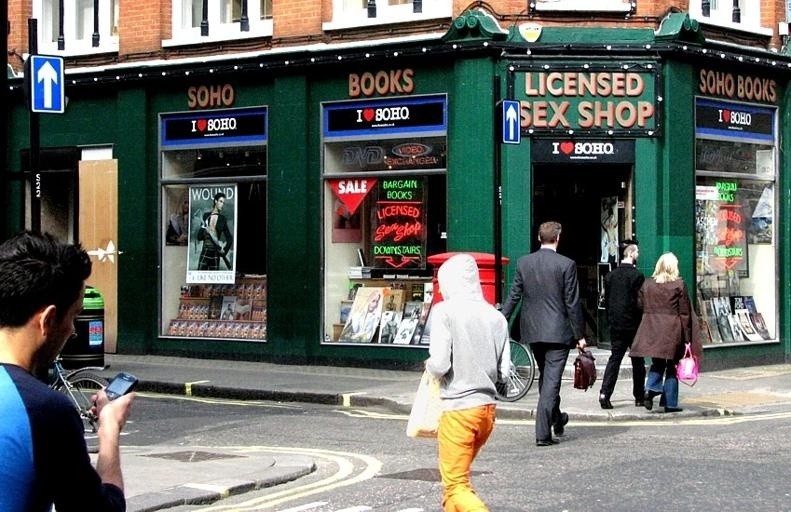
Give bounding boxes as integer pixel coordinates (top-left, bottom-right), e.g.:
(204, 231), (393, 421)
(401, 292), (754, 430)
(597, 239), (647, 408)
(499, 222), (587, 448)
(424, 252), (512, 512)
(197, 193), (233, 269)
(626, 249), (691, 413)
(0, 226), (137, 512)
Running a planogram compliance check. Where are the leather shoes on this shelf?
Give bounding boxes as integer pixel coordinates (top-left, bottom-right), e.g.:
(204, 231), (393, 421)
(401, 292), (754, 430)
(664, 407), (683, 412)
(600, 393), (614, 409)
(536, 438), (560, 445)
(553, 412), (569, 436)
(644, 389), (653, 410)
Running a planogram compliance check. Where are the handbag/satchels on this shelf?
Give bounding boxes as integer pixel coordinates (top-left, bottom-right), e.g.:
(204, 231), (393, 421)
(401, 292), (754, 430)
(573, 350), (597, 390)
(676, 346), (698, 380)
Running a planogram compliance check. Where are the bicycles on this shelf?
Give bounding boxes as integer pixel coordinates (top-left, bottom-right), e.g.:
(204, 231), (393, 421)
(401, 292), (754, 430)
(492, 338), (537, 402)
(44, 351), (111, 453)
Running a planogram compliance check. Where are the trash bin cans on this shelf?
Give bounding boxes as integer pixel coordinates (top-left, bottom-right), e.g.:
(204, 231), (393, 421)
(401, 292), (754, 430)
(427, 251), (510, 306)
(59, 286), (104, 372)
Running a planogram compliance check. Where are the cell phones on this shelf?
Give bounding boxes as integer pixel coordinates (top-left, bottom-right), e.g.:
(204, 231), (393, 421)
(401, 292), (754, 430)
(94, 372), (139, 412)
